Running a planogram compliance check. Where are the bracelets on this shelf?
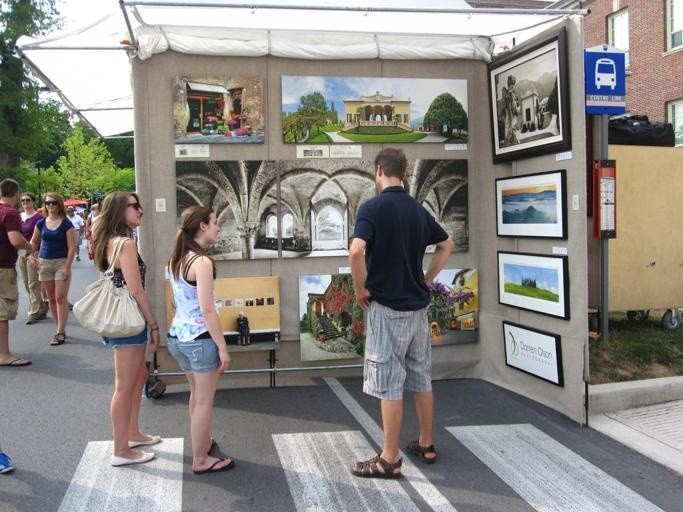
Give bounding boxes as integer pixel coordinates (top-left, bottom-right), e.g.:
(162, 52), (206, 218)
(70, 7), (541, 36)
(152, 326), (159, 331)
(149, 322), (157, 327)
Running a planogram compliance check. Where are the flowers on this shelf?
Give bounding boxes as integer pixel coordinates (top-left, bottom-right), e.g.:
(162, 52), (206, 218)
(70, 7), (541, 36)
(428, 280), (474, 307)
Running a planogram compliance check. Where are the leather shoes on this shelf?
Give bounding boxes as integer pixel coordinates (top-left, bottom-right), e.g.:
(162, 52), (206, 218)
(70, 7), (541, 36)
(128, 435), (160, 448)
(111, 452), (155, 466)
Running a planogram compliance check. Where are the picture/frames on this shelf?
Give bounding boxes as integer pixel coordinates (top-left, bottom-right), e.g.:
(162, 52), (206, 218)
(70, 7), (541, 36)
(492, 169), (570, 243)
(496, 248), (572, 322)
(500, 318), (565, 388)
(485, 26), (572, 168)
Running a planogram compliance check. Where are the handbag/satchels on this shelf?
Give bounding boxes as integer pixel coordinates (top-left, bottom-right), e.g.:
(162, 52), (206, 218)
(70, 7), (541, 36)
(609, 114), (674, 146)
(70, 269), (146, 339)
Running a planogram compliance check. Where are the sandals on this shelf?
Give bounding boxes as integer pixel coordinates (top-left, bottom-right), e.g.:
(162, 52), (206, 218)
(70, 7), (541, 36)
(407, 439), (437, 463)
(351, 453), (402, 479)
(50, 332), (65, 345)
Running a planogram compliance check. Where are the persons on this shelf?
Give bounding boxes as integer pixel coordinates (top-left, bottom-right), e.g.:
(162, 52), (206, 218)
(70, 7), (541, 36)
(167, 206), (234, 475)
(0, 179), (31, 366)
(94, 191), (161, 466)
(29, 194), (76, 345)
(17, 193), (44, 324)
(34, 206), (100, 310)
(236, 313), (251, 345)
(349, 149), (454, 479)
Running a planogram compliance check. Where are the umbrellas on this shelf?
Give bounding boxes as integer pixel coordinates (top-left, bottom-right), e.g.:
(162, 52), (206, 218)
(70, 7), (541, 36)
(64, 198), (89, 205)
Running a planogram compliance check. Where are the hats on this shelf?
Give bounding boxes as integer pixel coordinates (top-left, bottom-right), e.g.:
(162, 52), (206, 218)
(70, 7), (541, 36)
(66, 206), (74, 211)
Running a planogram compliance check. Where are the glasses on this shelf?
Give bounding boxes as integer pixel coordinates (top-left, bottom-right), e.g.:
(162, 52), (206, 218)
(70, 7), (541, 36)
(45, 201), (57, 205)
(21, 198), (31, 202)
(127, 202), (139, 211)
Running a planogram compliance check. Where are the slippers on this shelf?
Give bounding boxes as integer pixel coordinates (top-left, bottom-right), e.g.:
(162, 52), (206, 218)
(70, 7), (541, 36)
(194, 458), (234, 474)
(0, 358), (31, 367)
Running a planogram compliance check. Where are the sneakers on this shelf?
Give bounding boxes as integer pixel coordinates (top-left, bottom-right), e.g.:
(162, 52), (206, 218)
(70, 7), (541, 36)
(26, 301), (48, 324)
(0, 450), (14, 474)
(67, 302), (73, 310)
(76, 257), (80, 261)
